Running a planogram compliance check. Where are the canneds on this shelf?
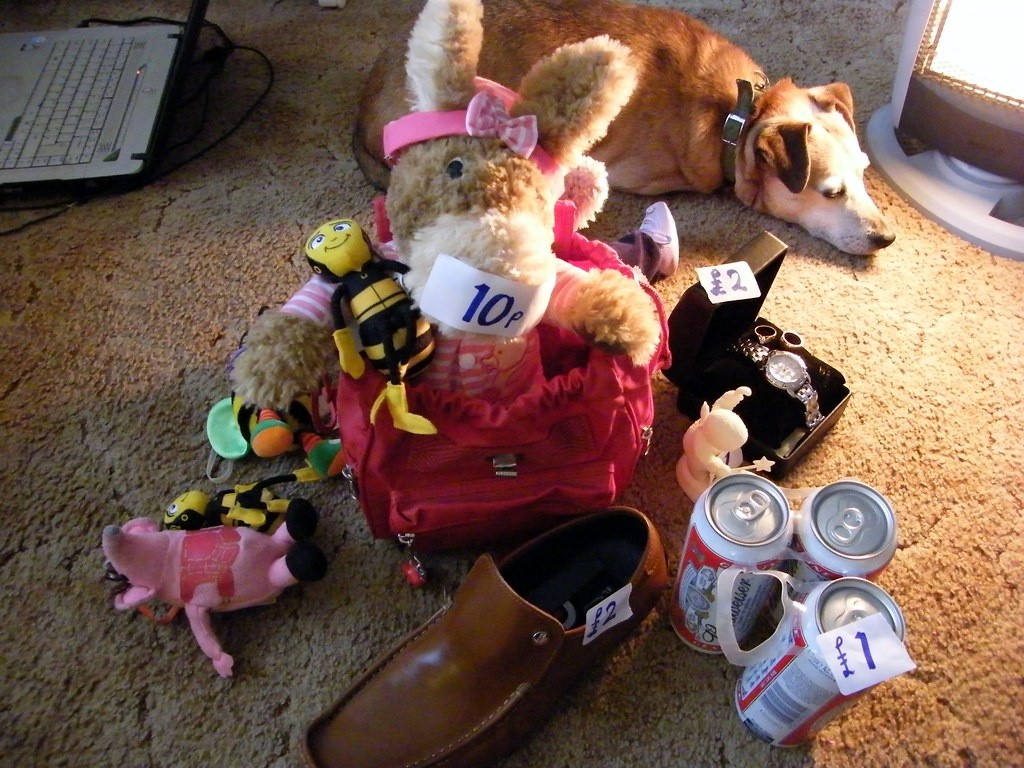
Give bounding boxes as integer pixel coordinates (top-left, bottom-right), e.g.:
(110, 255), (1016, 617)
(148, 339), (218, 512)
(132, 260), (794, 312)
(670, 471), (906, 749)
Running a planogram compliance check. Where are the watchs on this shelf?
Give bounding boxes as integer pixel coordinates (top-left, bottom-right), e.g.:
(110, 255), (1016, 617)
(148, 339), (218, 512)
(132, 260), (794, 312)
(725, 336), (829, 430)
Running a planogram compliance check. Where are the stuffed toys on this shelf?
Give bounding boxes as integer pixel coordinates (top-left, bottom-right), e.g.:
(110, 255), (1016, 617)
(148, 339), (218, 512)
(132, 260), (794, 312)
(101, 496), (325, 677)
(159, 482), (294, 536)
(231, 0), (681, 482)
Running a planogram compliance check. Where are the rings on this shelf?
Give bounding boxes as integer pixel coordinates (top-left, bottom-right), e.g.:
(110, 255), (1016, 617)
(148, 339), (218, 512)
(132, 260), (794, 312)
(753, 325), (778, 344)
(778, 332), (807, 351)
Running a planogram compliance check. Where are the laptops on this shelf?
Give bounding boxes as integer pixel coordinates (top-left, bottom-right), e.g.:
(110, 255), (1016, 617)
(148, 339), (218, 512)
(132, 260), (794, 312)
(0, 0), (212, 201)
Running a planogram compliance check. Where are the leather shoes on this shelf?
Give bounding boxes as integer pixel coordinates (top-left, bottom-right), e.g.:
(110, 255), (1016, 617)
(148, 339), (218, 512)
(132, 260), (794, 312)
(301, 506), (669, 768)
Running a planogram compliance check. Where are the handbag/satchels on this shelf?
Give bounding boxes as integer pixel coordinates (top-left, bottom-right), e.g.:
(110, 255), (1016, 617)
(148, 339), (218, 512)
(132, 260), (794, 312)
(338, 195), (672, 544)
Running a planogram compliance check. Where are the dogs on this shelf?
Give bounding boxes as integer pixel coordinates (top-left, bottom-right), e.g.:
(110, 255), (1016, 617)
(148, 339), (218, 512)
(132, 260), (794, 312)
(352, 1), (897, 258)
